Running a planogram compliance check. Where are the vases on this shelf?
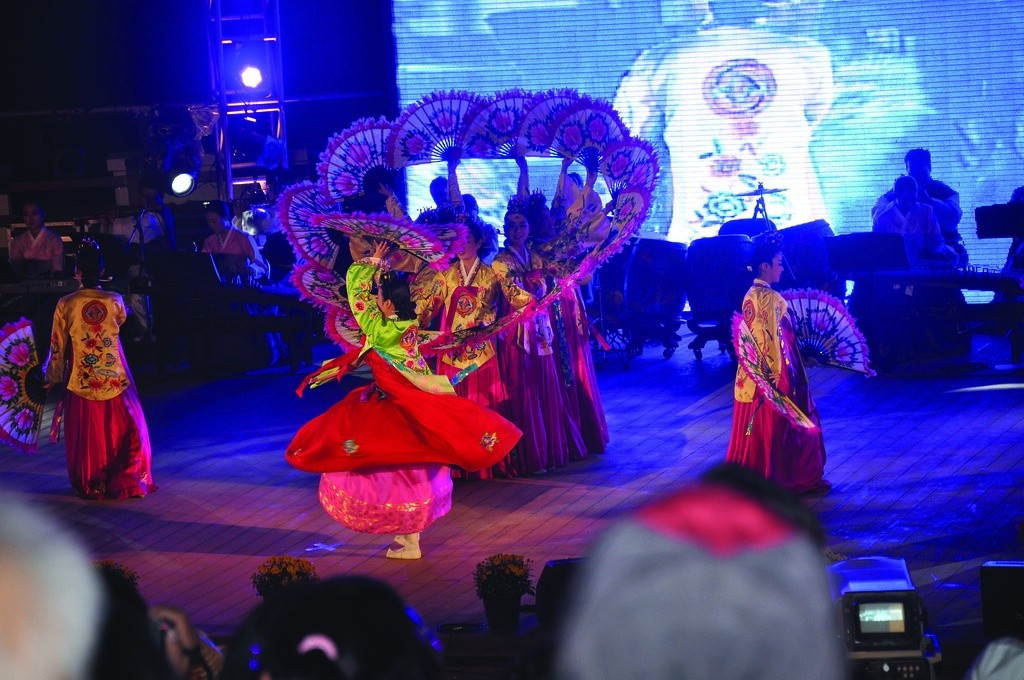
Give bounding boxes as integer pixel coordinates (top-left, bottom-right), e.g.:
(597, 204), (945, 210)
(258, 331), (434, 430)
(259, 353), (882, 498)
(482, 595), (521, 634)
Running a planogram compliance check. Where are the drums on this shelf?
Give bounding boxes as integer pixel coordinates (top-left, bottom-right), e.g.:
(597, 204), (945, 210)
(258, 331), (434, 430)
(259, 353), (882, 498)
(765, 218), (847, 308)
(718, 217), (778, 241)
(686, 233), (751, 320)
(590, 231), (688, 319)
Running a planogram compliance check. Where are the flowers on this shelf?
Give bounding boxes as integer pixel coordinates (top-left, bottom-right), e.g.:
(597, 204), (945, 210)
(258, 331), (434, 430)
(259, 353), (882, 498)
(472, 552), (537, 599)
(92, 560), (141, 588)
(250, 553), (319, 600)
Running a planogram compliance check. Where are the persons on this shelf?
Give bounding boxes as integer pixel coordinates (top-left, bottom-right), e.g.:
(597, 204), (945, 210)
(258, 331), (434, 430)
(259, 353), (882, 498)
(0, 495), (221, 680)
(309, 119), (657, 480)
(609, 0), (836, 241)
(989, 185), (1024, 304)
(286, 237), (523, 559)
(553, 462), (848, 679)
(42, 237), (160, 500)
(8, 199), (66, 274)
(221, 572), (448, 680)
(871, 149), (969, 268)
(726, 247), (830, 496)
(199, 200), (256, 258)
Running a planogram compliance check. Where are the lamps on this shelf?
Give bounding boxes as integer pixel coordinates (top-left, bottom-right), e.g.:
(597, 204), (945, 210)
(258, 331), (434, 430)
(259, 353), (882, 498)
(161, 156), (195, 198)
(238, 39), (265, 90)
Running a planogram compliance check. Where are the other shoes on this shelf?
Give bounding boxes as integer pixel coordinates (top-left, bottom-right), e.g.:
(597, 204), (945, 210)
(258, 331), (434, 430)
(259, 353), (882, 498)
(386, 534), (422, 560)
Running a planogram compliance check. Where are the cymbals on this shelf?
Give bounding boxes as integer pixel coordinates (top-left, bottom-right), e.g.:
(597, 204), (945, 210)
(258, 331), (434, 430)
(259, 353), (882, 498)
(734, 188), (788, 198)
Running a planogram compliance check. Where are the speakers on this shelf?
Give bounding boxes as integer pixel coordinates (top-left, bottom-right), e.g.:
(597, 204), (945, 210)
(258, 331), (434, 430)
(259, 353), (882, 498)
(980, 561), (1024, 639)
(536, 557), (591, 628)
